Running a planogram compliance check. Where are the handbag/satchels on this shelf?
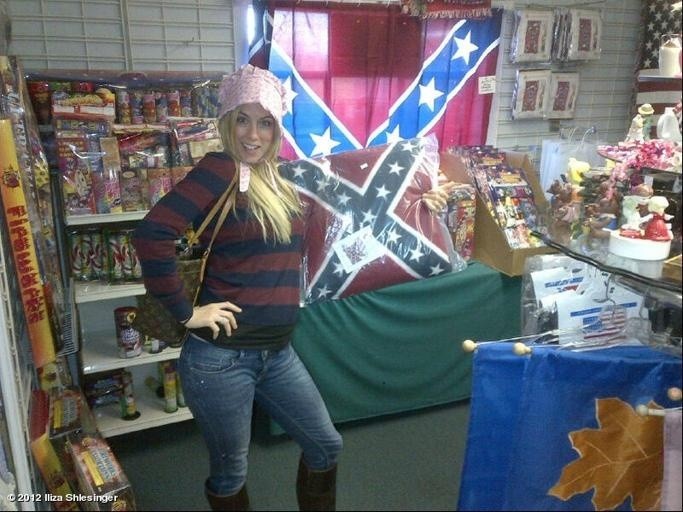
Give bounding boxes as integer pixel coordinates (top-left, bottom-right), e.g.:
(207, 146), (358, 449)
(131, 238), (207, 349)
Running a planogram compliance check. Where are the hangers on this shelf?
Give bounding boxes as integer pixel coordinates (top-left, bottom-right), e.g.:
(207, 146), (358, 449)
(462, 260), (683, 357)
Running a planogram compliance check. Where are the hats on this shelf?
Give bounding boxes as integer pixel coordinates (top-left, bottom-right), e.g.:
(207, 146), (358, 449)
(218, 64), (286, 136)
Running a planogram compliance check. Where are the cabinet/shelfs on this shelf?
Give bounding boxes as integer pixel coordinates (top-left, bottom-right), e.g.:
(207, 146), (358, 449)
(65, 211), (196, 440)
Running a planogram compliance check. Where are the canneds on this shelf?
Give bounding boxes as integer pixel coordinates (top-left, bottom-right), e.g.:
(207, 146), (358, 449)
(113, 306), (165, 358)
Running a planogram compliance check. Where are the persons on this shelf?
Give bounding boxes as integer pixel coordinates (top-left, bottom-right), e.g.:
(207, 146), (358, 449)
(133, 63), (457, 511)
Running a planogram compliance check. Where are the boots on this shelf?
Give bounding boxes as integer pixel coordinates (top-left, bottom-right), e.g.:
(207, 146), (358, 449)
(296, 453), (338, 512)
(204, 476), (253, 512)
